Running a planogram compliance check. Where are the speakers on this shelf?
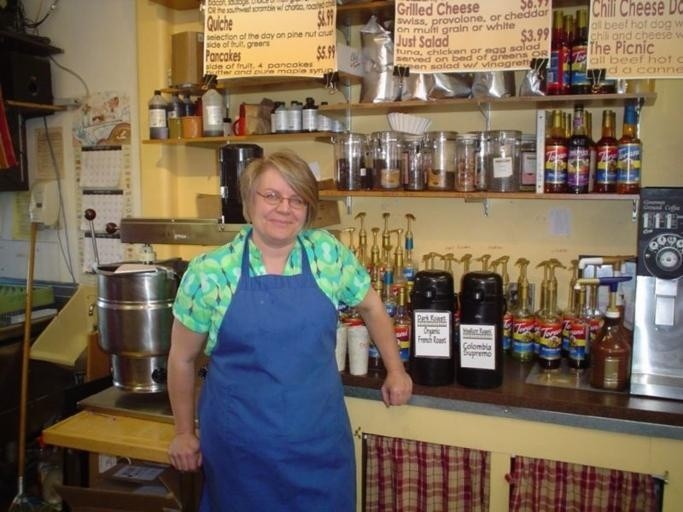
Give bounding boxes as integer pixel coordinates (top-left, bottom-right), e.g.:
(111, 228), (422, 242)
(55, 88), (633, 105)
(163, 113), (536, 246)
(0, 50), (53, 105)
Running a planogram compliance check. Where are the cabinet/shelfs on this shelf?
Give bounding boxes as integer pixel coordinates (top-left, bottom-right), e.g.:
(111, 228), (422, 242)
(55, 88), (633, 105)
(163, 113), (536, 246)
(134, 0), (659, 202)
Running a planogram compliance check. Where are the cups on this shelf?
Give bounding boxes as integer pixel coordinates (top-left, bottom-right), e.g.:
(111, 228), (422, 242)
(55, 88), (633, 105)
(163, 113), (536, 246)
(336, 325), (369, 376)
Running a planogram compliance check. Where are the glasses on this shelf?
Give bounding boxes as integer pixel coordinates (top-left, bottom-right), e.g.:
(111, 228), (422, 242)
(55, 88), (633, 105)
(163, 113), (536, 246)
(255, 189), (305, 209)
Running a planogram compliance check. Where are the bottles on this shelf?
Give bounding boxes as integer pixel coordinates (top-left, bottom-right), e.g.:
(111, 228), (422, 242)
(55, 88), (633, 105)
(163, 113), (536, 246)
(334, 129), (537, 194)
(544, 9), (643, 193)
(149, 84), (346, 140)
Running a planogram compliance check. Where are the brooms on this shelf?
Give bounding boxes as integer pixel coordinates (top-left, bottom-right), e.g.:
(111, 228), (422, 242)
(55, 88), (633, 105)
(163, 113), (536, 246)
(6, 223), (66, 512)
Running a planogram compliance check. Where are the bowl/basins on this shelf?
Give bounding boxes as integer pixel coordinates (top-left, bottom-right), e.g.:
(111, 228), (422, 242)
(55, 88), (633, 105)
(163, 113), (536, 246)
(387, 111), (431, 129)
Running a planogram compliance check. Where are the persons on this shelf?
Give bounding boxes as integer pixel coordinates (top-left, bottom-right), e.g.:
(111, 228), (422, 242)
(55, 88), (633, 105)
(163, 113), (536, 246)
(166, 147), (412, 512)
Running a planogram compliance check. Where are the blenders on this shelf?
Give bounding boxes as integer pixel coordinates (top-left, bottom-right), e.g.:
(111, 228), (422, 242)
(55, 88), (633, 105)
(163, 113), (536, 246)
(219, 143), (263, 224)
